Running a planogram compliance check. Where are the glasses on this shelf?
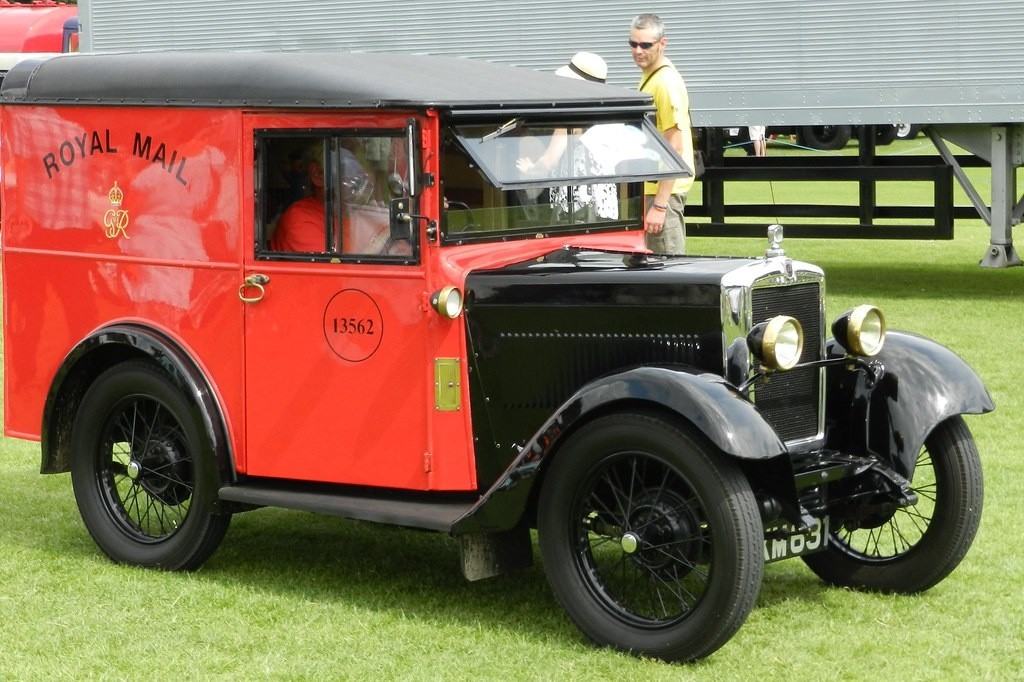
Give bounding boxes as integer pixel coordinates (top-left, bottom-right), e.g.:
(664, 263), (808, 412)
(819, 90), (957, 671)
(628, 38), (660, 49)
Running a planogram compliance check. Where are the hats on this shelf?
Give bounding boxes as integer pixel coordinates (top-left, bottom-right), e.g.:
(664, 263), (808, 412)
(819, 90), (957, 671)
(555, 51), (608, 84)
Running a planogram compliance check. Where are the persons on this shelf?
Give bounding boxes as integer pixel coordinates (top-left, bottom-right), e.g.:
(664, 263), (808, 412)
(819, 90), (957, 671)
(749, 126), (766, 156)
(628, 13), (696, 255)
(268, 134), (413, 255)
(516, 51), (618, 226)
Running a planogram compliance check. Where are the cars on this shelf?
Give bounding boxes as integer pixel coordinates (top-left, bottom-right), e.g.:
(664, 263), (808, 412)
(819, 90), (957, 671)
(0, 51), (997, 667)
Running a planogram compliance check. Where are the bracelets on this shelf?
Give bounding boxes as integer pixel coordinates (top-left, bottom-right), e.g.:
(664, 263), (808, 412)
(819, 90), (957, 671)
(653, 203), (666, 212)
